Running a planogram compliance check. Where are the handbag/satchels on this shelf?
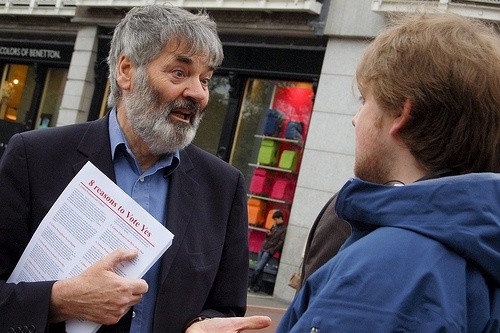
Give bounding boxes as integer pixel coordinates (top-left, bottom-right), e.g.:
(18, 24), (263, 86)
(288, 263), (302, 289)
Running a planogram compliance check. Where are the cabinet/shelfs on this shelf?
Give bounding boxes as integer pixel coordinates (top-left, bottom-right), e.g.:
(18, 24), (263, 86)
(246, 135), (303, 233)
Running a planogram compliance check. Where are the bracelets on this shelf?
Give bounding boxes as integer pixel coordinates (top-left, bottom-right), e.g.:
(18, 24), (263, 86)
(184, 315), (208, 332)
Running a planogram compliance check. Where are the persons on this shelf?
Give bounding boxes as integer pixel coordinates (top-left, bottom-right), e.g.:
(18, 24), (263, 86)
(247, 210), (287, 292)
(275, 12), (500, 333)
(2, 6), (271, 333)
